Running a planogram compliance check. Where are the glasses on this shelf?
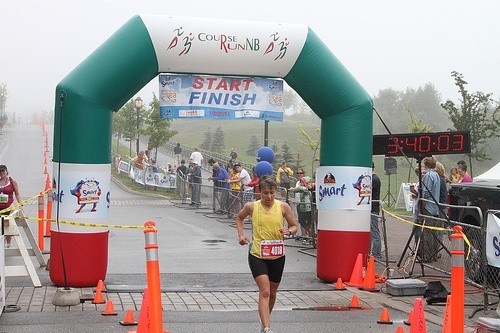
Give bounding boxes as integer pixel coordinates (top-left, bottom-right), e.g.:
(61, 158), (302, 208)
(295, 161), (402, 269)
(297, 172), (304, 174)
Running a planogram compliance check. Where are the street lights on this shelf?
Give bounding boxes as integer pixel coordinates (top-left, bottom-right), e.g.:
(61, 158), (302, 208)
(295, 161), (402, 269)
(134, 96), (143, 156)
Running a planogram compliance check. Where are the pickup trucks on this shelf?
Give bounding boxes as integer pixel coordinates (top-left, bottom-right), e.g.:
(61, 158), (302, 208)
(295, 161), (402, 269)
(446, 182), (500, 284)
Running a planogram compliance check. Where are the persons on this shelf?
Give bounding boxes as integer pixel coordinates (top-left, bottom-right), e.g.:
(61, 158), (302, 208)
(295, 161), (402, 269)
(290, 168), (318, 244)
(276, 161), (294, 201)
(133, 144), (159, 191)
(208, 148), (262, 222)
(177, 159), (202, 206)
(371, 161), (381, 261)
(188, 147), (204, 167)
(173, 143), (181, 166)
(237, 175), (298, 333)
(412, 155), (473, 262)
(0, 165), (24, 248)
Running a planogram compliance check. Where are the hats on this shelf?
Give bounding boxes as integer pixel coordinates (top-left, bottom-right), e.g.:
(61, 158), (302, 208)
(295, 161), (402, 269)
(234, 162), (241, 166)
(300, 176), (311, 187)
(0, 165), (7, 171)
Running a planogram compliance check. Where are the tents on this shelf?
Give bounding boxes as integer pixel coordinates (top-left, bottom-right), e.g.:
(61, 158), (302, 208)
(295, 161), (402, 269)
(472, 162), (500, 183)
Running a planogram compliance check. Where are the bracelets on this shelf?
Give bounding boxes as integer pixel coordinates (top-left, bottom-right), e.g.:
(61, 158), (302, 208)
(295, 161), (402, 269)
(21, 205), (23, 206)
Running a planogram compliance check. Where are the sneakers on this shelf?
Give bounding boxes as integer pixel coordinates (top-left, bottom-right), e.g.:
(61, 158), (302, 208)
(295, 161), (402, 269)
(259, 324), (273, 333)
(4, 243), (11, 248)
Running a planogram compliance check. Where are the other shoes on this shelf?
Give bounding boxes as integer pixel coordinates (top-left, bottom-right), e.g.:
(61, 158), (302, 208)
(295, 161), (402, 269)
(415, 252), (441, 263)
(215, 210), (228, 215)
(189, 202), (195, 205)
(298, 233), (313, 242)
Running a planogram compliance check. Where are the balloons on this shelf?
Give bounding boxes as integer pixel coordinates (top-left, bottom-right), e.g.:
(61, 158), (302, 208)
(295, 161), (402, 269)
(255, 146), (275, 163)
(254, 161), (273, 178)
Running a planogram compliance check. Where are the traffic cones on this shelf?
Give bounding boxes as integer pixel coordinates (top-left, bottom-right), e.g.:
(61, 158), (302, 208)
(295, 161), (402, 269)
(395, 326), (405, 333)
(91, 289), (106, 304)
(127, 288), (170, 333)
(101, 299), (119, 316)
(42, 124), (51, 194)
(94, 280), (107, 292)
(348, 295), (362, 309)
(410, 297), (427, 333)
(344, 253), (364, 287)
(334, 278), (347, 290)
(119, 307), (138, 326)
(376, 308), (394, 325)
(403, 309), (413, 326)
(358, 256), (381, 292)
(441, 294), (451, 333)
